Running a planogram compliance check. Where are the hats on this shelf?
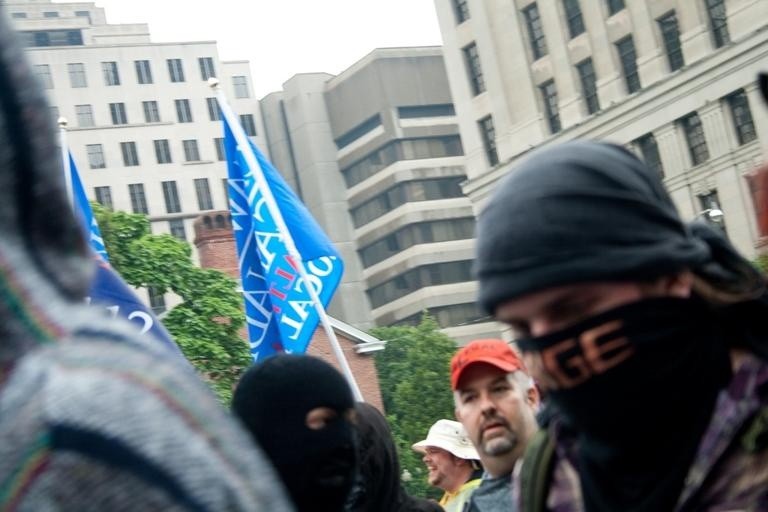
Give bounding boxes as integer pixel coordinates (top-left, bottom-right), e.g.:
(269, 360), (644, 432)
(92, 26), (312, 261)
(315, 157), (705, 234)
(409, 417), (483, 463)
(448, 338), (530, 392)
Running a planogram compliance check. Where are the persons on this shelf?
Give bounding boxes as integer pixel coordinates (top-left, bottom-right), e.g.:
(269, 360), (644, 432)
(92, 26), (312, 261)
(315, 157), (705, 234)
(231, 354), (355, 511)
(412, 418), (482, 512)
(471, 142), (768, 512)
(349, 403), (445, 511)
(450, 338), (549, 512)
(3, 13), (301, 512)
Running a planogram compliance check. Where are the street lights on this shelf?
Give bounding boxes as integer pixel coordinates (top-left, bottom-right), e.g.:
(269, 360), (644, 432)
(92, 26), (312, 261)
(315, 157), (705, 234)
(690, 203), (726, 226)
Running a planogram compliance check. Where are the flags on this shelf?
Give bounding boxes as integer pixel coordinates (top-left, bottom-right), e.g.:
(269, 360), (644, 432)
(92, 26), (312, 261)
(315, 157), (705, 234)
(91, 252), (189, 366)
(64, 141), (109, 263)
(214, 86), (343, 359)
